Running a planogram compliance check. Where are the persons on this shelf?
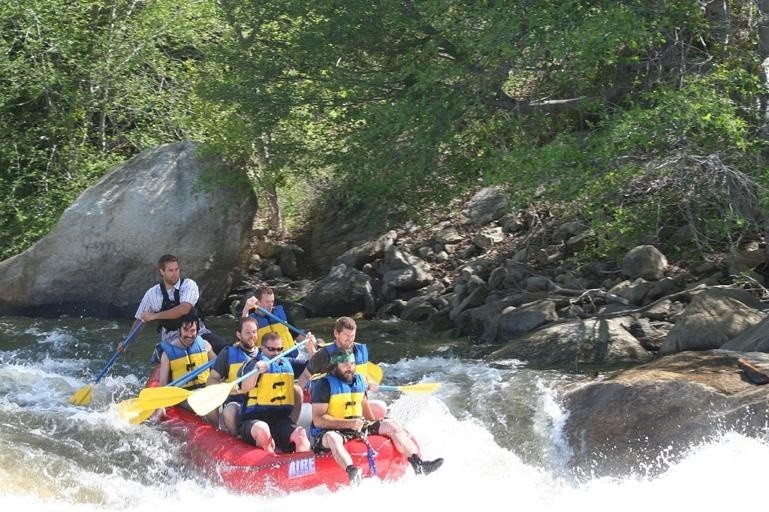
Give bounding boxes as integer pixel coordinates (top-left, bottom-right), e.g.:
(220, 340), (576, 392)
(308, 348), (444, 487)
(237, 331), (317, 453)
(206, 317), (304, 437)
(307, 316), (378, 398)
(242, 287), (324, 358)
(116, 255), (232, 362)
(151, 313), (223, 425)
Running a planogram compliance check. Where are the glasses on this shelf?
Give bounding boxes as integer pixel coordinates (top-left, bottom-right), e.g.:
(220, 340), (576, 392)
(268, 347), (283, 351)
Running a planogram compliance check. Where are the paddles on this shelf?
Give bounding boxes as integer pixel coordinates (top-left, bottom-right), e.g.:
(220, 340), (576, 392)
(115, 356), (216, 426)
(139, 386), (192, 409)
(188, 338), (309, 416)
(378, 383), (437, 392)
(251, 305), (382, 384)
(70, 319), (144, 406)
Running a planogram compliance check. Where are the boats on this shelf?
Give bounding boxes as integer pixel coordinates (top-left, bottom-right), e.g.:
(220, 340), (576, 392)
(145, 357), (426, 496)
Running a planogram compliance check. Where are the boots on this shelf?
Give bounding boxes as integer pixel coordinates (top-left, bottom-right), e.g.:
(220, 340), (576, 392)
(346, 465), (358, 480)
(408, 454), (443, 474)
